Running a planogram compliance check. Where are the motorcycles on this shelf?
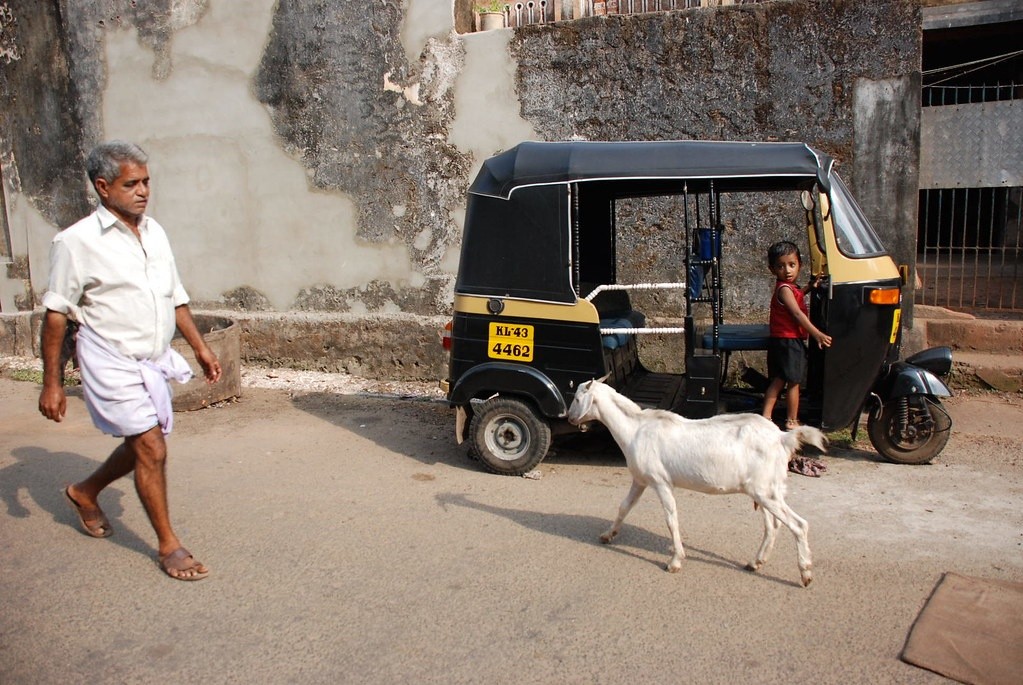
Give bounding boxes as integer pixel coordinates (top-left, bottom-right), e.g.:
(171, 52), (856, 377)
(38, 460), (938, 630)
(439, 138), (955, 480)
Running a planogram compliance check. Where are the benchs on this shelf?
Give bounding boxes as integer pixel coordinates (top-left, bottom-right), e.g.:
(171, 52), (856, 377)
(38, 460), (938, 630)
(577, 272), (646, 393)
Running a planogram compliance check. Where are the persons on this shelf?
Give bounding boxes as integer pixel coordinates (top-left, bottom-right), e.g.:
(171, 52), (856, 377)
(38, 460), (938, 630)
(762, 241), (832, 431)
(38, 141), (222, 580)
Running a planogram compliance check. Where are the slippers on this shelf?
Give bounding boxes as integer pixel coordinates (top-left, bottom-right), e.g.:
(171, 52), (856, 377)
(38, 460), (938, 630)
(159, 546), (210, 580)
(63, 483), (114, 537)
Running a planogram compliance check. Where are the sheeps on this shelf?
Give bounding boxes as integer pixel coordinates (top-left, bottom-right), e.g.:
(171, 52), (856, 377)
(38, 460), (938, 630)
(568, 370), (828, 587)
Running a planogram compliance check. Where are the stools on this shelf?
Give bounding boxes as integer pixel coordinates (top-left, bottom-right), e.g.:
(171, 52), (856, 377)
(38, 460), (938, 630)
(703, 321), (772, 398)
